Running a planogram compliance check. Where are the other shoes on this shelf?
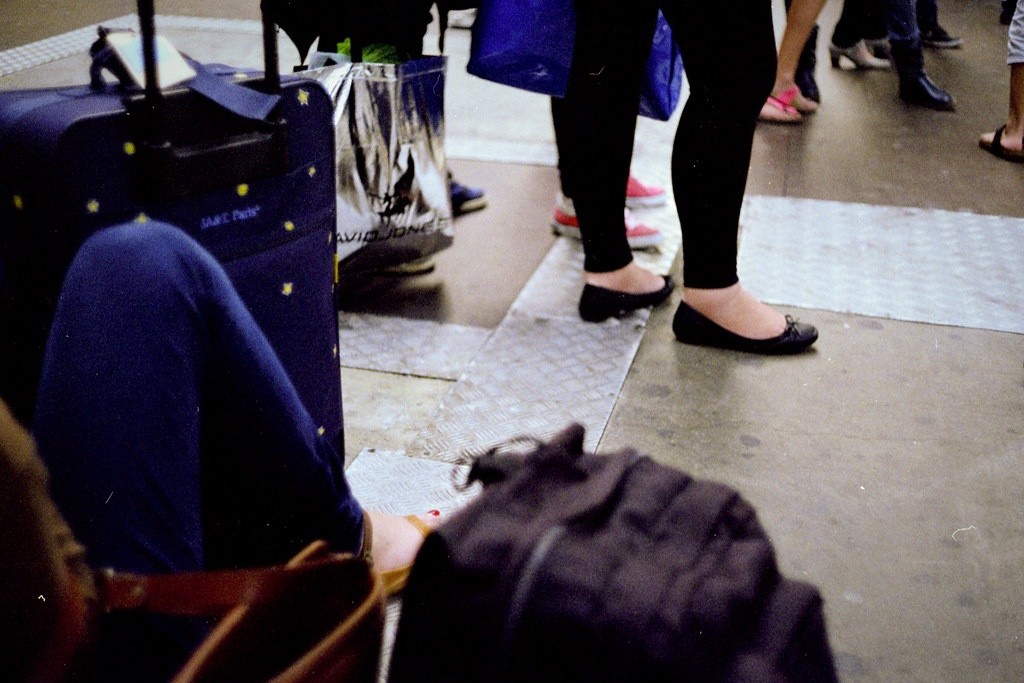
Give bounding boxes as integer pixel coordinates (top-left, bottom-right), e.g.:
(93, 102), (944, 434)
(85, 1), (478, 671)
(578, 273), (674, 323)
(751, 81), (818, 127)
(671, 301), (821, 357)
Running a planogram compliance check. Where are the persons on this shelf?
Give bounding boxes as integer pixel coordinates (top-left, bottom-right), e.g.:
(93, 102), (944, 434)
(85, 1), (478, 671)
(465, 0), (825, 353)
(830, 0), (965, 110)
(259, 0), (488, 274)
(0, 217), (447, 683)
(979, 0), (1024, 161)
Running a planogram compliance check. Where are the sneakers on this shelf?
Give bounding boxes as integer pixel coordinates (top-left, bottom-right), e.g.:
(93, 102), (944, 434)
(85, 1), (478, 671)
(547, 171), (663, 250)
(919, 25), (963, 48)
(448, 175), (489, 219)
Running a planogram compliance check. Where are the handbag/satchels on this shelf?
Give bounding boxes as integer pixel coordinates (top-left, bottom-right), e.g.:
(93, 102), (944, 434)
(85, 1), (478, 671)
(261, 54), (455, 292)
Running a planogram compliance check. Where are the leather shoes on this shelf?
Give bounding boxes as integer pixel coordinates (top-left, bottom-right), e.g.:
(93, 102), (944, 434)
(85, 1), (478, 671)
(898, 72), (957, 110)
(793, 68), (821, 101)
(826, 36), (891, 71)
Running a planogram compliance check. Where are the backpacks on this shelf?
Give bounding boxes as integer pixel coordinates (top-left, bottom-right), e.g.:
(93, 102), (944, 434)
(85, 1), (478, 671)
(386, 425), (839, 683)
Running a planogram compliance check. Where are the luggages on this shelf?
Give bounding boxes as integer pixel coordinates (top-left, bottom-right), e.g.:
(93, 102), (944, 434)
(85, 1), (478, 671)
(2, 0), (347, 478)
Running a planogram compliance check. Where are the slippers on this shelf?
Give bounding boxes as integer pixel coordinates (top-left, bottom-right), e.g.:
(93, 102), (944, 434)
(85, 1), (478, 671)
(979, 123), (1024, 165)
